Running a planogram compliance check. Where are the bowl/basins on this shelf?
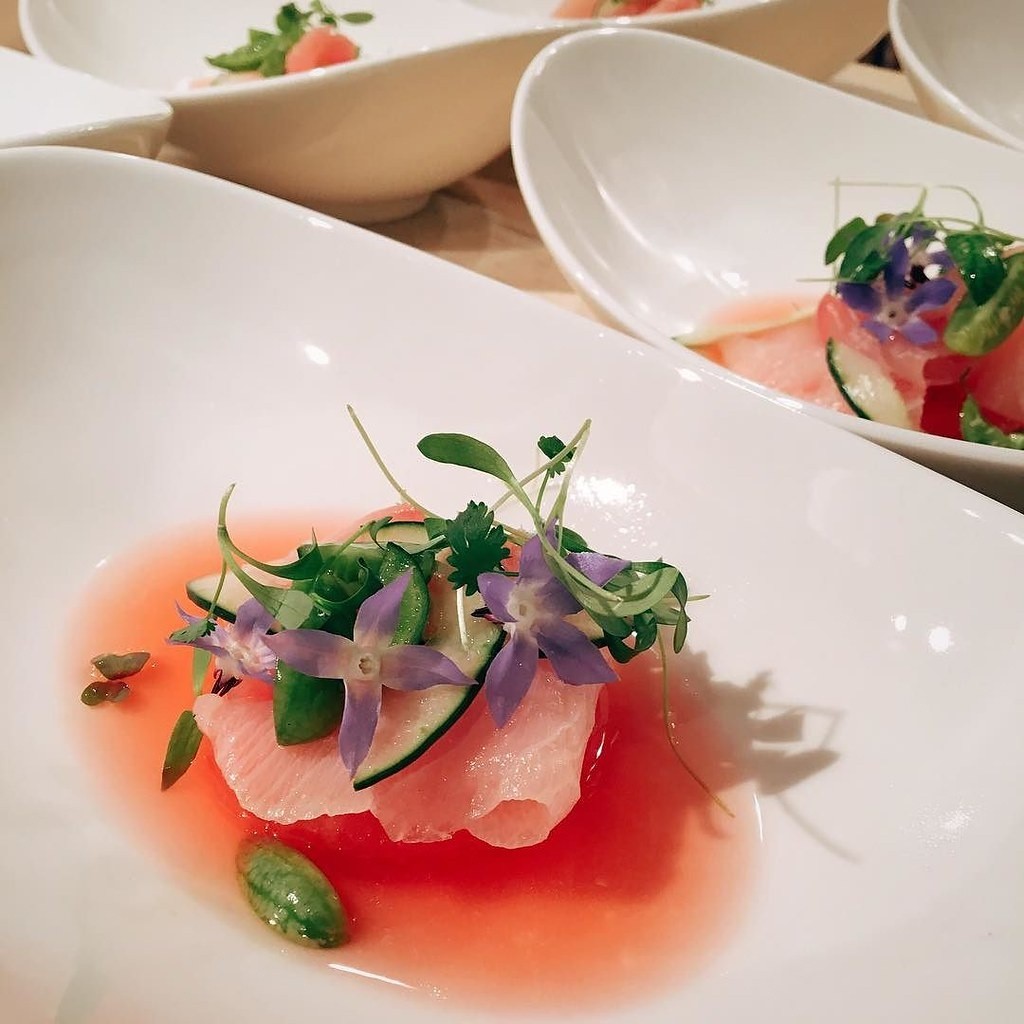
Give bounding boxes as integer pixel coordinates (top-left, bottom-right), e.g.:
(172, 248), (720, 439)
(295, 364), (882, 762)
(507, 27), (1024, 493)
(16, 0), (568, 223)
(562, 0), (895, 82)
(1, 45), (176, 159)
(539, 0), (889, 84)
(0, 139), (1024, 1018)
(884, 1), (1023, 148)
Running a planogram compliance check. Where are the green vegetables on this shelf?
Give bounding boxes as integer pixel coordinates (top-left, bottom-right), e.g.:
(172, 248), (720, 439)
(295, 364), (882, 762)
(800, 176), (1024, 452)
(84, 403), (707, 952)
(203, 1), (374, 77)
